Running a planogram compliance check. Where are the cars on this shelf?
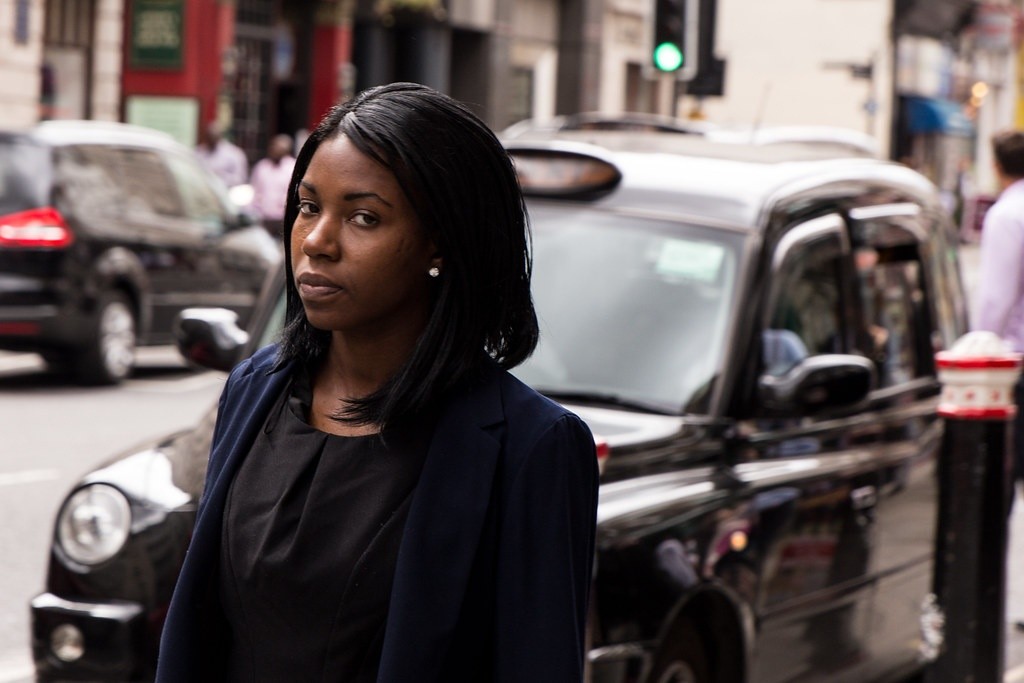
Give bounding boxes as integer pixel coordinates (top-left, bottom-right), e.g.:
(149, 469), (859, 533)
(0, 116), (282, 383)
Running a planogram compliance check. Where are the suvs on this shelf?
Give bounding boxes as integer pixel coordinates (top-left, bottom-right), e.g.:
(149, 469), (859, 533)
(21, 108), (981, 683)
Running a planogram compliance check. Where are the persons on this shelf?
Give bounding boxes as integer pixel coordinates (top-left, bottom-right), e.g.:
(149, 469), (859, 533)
(155, 82), (600, 683)
(191, 95), (298, 268)
(764, 133), (1024, 634)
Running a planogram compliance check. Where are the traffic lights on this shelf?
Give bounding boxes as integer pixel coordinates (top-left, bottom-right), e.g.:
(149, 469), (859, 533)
(649, 1), (696, 83)
